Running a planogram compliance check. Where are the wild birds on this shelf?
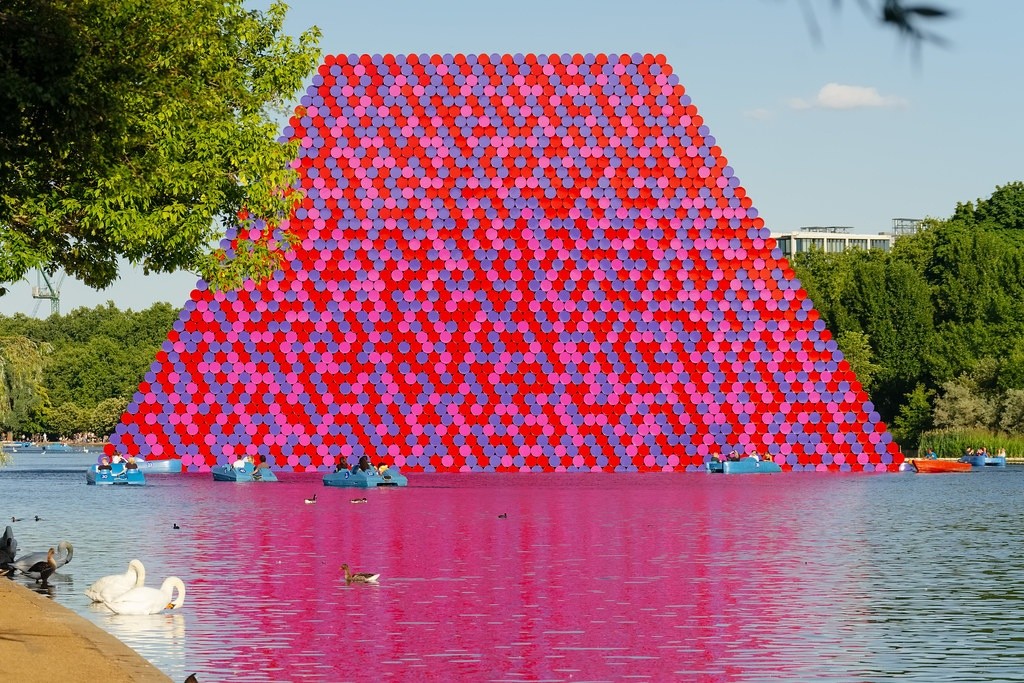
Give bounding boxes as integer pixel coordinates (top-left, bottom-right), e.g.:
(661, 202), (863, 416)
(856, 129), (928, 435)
(10, 446), (90, 454)
(11, 515), (42, 522)
(880, 0), (946, 38)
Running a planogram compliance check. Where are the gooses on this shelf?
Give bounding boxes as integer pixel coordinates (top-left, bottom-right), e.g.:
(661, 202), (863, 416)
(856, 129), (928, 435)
(340, 564), (381, 582)
(350, 497), (367, 503)
(84, 558), (185, 614)
(173, 523), (180, 529)
(0, 526), (74, 586)
(304, 494), (317, 503)
(498, 513), (507, 518)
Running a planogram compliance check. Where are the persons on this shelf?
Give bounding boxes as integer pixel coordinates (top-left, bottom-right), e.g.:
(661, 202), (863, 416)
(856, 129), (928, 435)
(966, 446), (1006, 458)
(923, 447), (936, 460)
(233, 454), (269, 474)
(97, 450), (139, 474)
(333, 456), (389, 478)
(711, 450), (773, 463)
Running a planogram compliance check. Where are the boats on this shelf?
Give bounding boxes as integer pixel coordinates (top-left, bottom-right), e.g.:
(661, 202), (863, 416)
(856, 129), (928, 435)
(86, 463), (144, 486)
(912, 459), (971, 473)
(322, 465), (408, 487)
(958, 455), (1005, 466)
(705, 458), (782, 474)
(211, 463), (278, 483)
(134, 459), (181, 475)
